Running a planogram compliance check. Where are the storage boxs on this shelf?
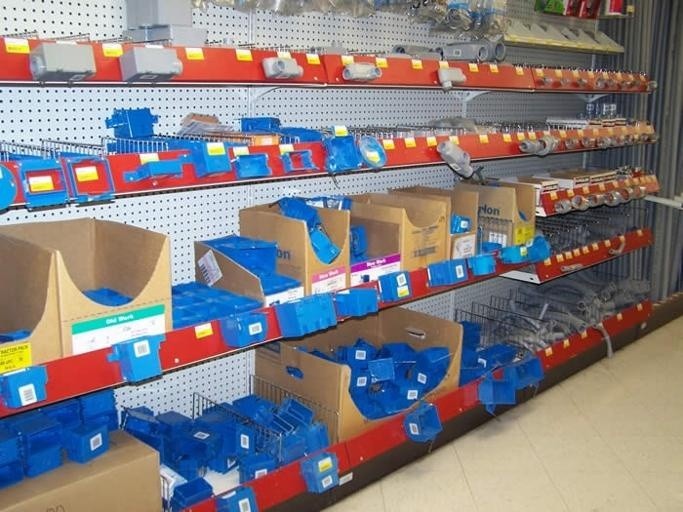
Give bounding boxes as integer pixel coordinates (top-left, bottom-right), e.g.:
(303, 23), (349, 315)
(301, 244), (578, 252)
(253, 306), (464, 445)
(0, 425), (164, 512)
(257, 306), (464, 447)
(3, 219), (174, 367)
(195, 178), (537, 300)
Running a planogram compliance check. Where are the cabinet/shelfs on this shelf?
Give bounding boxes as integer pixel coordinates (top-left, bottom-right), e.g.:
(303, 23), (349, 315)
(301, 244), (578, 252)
(453, 0), (661, 423)
(0, 1), (541, 512)
